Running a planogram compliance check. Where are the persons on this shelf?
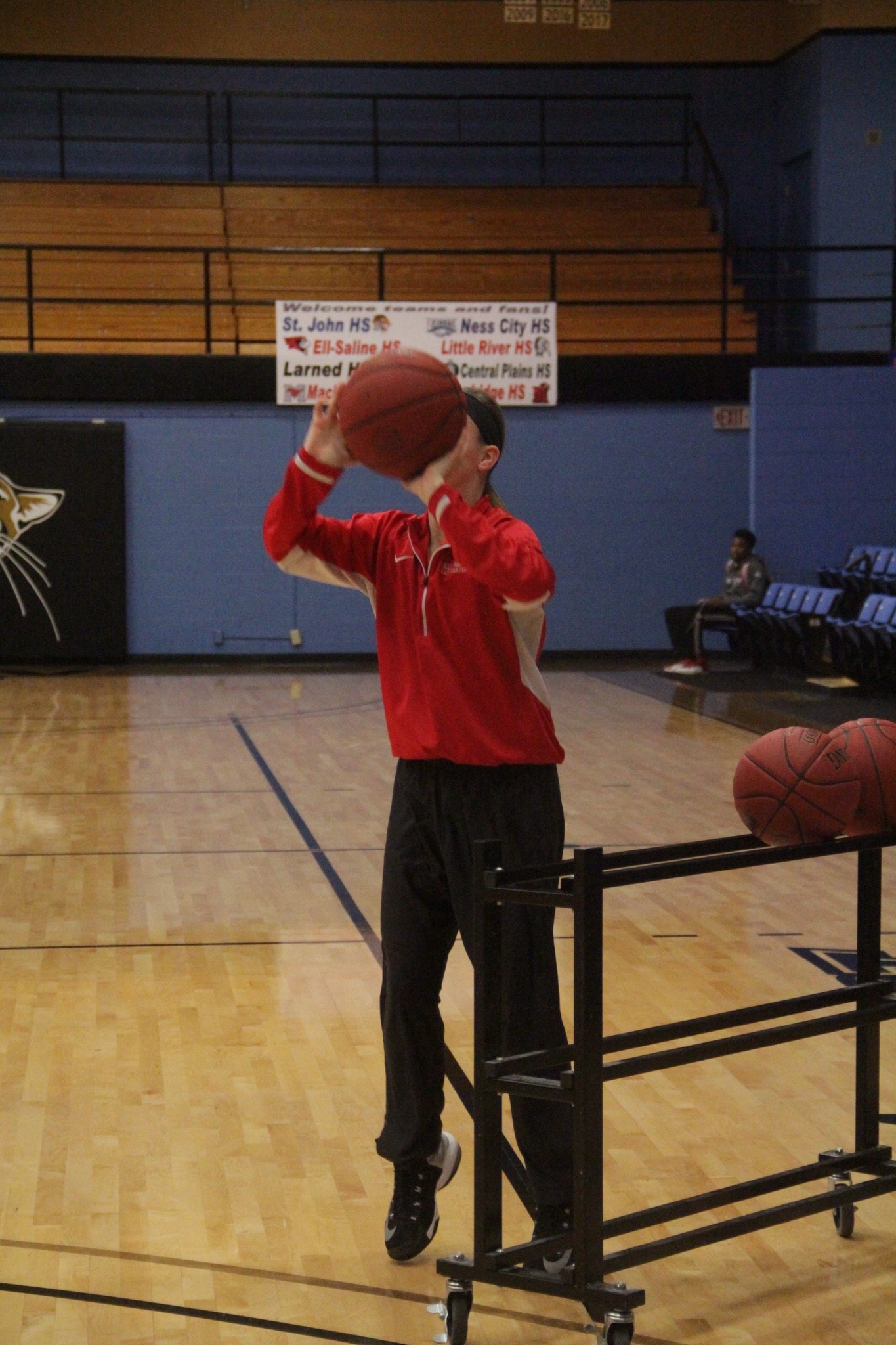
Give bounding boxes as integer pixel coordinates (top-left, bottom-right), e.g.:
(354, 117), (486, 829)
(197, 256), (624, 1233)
(663, 527), (770, 674)
(264, 385), (579, 1272)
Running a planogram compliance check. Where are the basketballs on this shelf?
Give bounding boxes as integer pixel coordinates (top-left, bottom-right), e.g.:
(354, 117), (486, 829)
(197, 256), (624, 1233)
(336, 346), (468, 482)
(826, 717), (896, 840)
(731, 725), (864, 847)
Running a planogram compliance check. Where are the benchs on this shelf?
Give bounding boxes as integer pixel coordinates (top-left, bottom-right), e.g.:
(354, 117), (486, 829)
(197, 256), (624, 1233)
(0, 179), (758, 357)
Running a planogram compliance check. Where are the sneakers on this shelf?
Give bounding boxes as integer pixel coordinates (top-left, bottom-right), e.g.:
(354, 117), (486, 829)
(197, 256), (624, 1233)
(663, 657), (692, 675)
(384, 1131), (462, 1262)
(539, 1201), (574, 1274)
(674, 658), (710, 677)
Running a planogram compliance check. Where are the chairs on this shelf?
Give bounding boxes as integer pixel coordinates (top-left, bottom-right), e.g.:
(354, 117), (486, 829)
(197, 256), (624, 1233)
(729, 546), (896, 688)
